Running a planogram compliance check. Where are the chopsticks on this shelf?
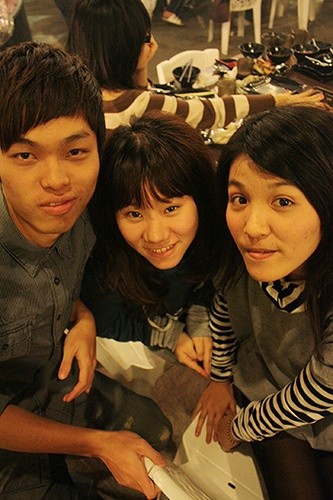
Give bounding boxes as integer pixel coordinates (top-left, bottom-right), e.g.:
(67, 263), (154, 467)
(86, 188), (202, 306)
(179, 58), (194, 82)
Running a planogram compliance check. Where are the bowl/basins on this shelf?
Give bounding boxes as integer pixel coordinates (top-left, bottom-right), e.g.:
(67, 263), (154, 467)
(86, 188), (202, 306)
(238, 42), (265, 59)
(213, 59), (236, 73)
(173, 66), (201, 88)
(261, 29), (333, 65)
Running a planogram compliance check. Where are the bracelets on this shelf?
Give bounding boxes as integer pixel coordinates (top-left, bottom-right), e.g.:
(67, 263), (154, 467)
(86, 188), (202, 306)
(227, 413), (240, 445)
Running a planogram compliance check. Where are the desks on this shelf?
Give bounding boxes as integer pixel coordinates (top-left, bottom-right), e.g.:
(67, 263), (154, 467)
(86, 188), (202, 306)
(171, 49), (333, 97)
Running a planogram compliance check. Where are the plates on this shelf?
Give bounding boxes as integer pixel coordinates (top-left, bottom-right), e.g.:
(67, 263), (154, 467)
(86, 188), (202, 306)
(240, 76), (301, 95)
(147, 72), (218, 98)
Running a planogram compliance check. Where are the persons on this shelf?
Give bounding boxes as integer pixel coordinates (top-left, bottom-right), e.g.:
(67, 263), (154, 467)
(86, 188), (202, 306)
(162, 0), (185, 25)
(82, 113), (219, 378)
(1, 40), (175, 499)
(188, 105), (333, 500)
(0, 0), (34, 51)
(64, 1), (329, 145)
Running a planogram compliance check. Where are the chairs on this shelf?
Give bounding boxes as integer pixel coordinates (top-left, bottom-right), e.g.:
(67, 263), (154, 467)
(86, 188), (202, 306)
(208, 0), (262, 58)
(156, 48), (218, 84)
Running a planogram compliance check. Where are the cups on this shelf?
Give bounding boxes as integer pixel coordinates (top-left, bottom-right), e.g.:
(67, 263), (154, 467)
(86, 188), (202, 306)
(217, 77), (236, 97)
(238, 57), (253, 77)
